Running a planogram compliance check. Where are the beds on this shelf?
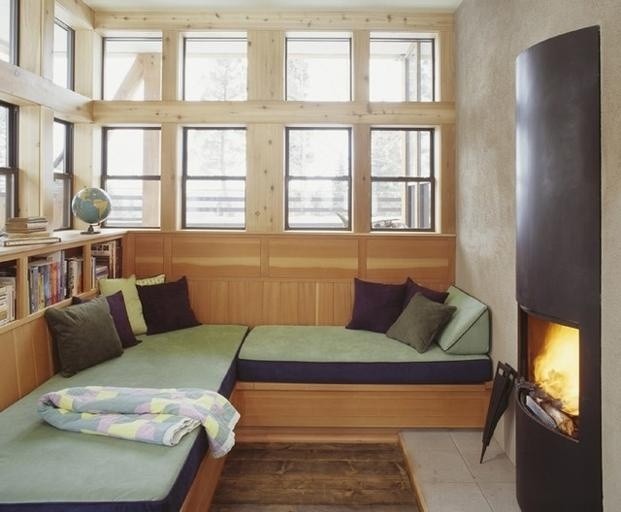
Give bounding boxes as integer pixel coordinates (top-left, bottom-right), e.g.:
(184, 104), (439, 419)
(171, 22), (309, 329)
(0, 325), (248, 512)
(234, 325), (489, 430)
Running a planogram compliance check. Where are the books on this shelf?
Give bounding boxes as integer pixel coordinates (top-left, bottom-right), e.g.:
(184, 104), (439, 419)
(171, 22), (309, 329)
(0, 218), (121, 328)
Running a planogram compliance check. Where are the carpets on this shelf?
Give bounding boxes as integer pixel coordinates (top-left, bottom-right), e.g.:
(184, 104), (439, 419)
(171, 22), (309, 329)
(206, 442), (425, 512)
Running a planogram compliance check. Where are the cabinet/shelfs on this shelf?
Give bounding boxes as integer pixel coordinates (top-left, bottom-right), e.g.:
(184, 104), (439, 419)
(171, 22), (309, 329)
(0, 227), (122, 411)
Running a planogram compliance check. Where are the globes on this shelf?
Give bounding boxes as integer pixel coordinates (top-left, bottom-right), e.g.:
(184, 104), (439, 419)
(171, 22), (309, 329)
(72, 187), (112, 234)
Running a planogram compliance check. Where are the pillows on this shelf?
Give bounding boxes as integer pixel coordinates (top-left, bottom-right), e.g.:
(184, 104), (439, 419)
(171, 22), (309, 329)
(45, 297), (123, 378)
(134, 273), (166, 287)
(98, 275), (138, 333)
(345, 275), (405, 332)
(133, 277), (200, 335)
(385, 292), (455, 352)
(403, 277), (447, 305)
(72, 291), (137, 348)
